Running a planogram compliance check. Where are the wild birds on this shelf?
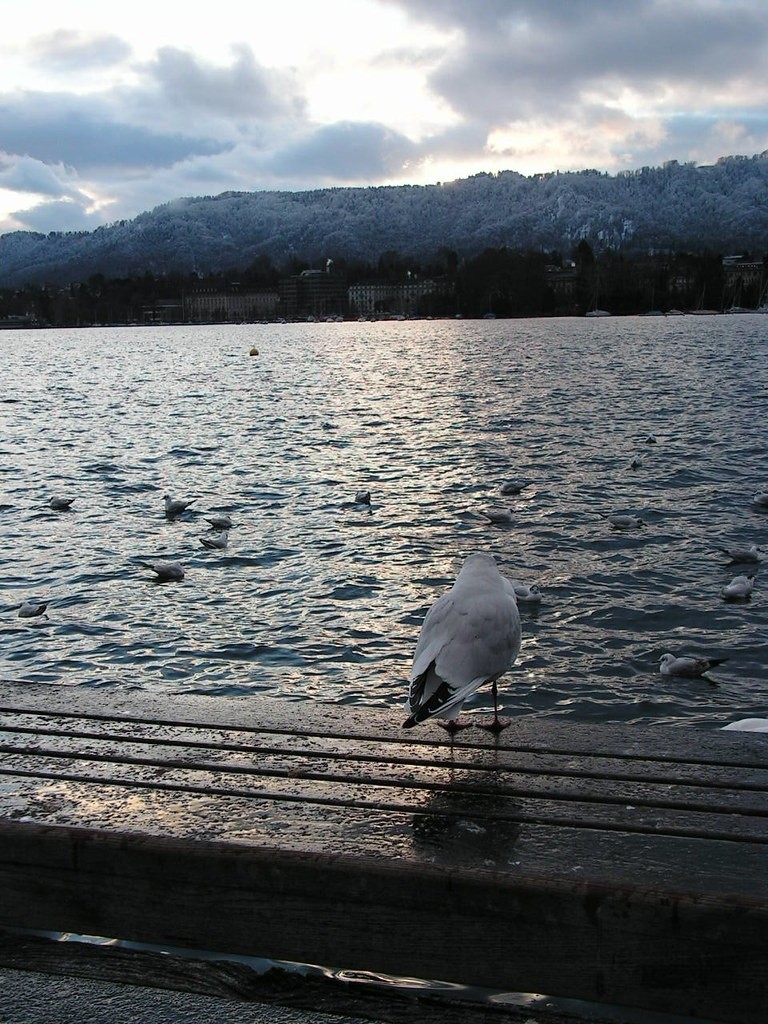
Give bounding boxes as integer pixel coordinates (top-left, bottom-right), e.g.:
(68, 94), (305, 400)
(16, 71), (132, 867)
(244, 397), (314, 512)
(477, 508), (516, 522)
(203, 515), (233, 528)
(497, 481), (534, 493)
(514, 584), (542, 601)
(751, 490), (768, 503)
(725, 545), (764, 563)
(49, 495), (75, 507)
(147, 563), (185, 578)
(199, 533), (228, 548)
(630, 453), (643, 470)
(646, 432), (656, 444)
(18, 600), (47, 618)
(721, 574), (756, 598)
(402, 553), (522, 734)
(161, 495), (198, 513)
(657, 653), (728, 677)
(607, 515), (650, 528)
(355, 490), (372, 504)
